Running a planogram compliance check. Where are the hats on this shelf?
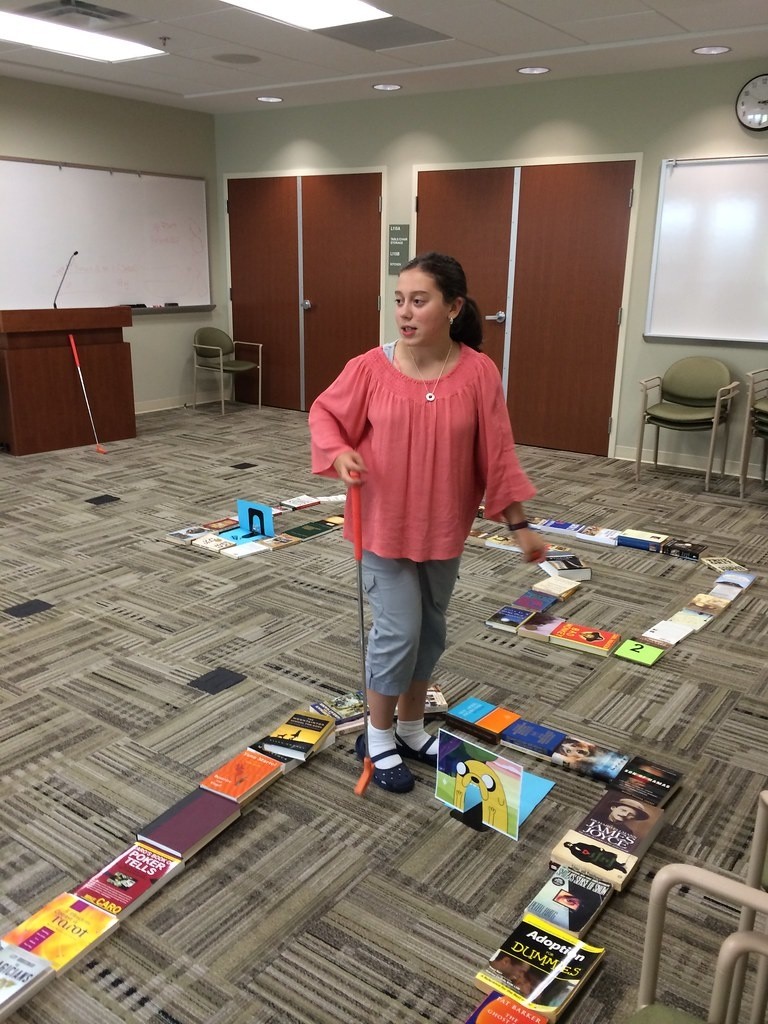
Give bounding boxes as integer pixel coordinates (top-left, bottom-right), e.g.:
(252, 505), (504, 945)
(609, 798), (649, 820)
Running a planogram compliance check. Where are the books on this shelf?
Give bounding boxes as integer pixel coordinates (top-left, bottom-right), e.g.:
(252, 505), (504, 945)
(165, 481), (754, 668)
(0, 671), (685, 1024)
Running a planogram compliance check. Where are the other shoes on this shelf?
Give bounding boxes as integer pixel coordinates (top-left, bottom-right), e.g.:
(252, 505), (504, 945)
(355, 733), (416, 794)
(392, 731), (436, 770)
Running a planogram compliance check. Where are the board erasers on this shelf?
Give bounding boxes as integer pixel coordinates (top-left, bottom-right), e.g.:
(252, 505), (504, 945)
(164, 302), (178, 308)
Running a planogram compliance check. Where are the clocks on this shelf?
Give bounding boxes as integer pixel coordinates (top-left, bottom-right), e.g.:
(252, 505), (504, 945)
(735, 74), (767, 131)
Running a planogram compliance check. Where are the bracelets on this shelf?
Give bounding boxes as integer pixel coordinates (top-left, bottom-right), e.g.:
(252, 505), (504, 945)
(508, 519), (528, 531)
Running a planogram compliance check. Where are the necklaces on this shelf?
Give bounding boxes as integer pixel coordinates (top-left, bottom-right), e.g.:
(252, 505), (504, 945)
(404, 339), (454, 401)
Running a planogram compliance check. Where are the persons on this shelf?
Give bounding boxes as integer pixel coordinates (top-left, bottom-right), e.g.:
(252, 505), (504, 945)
(307, 249), (550, 792)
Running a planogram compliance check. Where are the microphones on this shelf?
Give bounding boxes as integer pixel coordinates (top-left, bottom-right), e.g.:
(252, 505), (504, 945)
(54, 251), (78, 309)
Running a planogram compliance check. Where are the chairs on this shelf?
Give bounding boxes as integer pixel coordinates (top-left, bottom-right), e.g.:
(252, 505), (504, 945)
(625, 791), (768, 1024)
(633, 354), (767, 499)
(191, 327), (262, 415)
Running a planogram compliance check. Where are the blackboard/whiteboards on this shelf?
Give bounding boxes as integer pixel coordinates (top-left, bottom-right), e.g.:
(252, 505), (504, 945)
(0, 155), (216, 315)
(642, 152), (768, 345)
